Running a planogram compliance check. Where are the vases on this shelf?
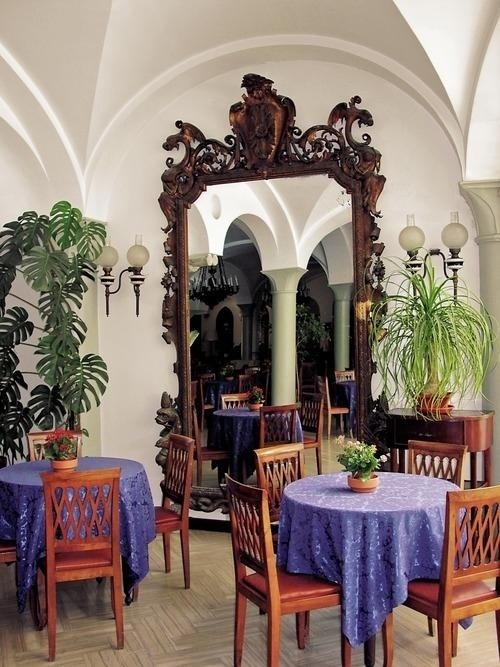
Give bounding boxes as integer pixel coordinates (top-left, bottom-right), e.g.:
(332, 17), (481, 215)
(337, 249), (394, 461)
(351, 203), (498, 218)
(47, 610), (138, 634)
(347, 473), (378, 493)
(51, 458), (78, 472)
(48, 455), (78, 472)
(347, 474), (379, 493)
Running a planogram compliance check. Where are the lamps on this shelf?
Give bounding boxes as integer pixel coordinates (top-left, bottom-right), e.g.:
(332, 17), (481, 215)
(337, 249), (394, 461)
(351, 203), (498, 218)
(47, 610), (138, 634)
(96, 234), (150, 317)
(398, 211), (469, 304)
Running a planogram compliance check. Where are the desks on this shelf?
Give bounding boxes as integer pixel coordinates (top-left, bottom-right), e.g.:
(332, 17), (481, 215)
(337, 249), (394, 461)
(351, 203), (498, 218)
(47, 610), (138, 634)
(276, 471), (470, 667)
(0, 457), (157, 602)
(385, 408), (495, 565)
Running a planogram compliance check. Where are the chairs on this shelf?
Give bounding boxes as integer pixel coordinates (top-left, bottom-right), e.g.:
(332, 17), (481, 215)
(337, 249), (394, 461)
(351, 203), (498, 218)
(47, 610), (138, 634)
(131, 433), (195, 602)
(408, 439), (469, 636)
(382, 485), (499, 667)
(190, 368), (356, 486)
(38, 466), (124, 662)
(0, 539), (43, 631)
(25, 431), (82, 461)
(252, 442), (310, 637)
(224, 473), (352, 667)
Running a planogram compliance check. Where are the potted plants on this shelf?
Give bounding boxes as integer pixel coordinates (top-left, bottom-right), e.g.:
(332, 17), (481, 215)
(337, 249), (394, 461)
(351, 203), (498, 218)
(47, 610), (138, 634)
(369, 246), (500, 421)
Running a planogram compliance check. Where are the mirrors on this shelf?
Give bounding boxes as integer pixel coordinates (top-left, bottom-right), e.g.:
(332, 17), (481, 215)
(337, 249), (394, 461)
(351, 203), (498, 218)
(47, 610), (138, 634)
(155, 74), (392, 515)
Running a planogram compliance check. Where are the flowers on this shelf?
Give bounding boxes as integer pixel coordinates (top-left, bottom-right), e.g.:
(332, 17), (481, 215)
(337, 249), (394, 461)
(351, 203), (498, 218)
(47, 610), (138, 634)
(44, 427), (78, 460)
(336, 435), (388, 482)
